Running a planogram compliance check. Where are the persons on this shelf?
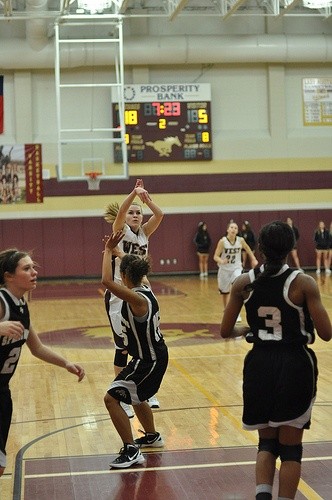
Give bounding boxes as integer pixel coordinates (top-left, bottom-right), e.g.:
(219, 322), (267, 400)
(240, 220), (256, 272)
(102, 231), (169, 469)
(220, 220), (332, 500)
(104, 179), (164, 419)
(0, 148), (21, 204)
(1, 248), (85, 480)
(213, 220), (258, 323)
(193, 221), (212, 277)
(314, 220), (331, 275)
(326, 223), (332, 273)
(282, 217), (304, 273)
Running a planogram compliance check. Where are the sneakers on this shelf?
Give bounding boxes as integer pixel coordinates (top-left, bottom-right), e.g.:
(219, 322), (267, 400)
(109, 443), (145, 468)
(133, 429), (164, 447)
(119, 401), (134, 418)
(146, 395), (160, 408)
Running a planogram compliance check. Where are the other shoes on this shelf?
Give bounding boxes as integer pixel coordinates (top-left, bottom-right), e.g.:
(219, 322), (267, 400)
(237, 314), (242, 322)
